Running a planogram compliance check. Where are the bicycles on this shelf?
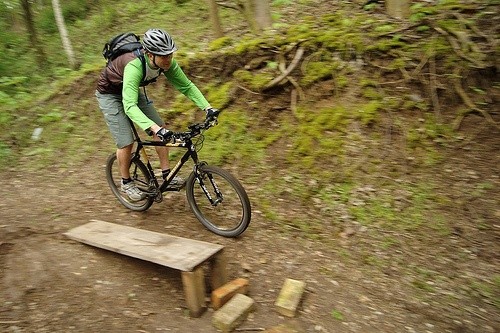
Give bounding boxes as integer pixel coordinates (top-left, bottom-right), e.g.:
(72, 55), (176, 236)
(105, 110), (251, 239)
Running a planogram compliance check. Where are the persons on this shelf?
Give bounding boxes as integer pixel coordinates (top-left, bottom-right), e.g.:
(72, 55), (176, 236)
(95, 28), (220, 202)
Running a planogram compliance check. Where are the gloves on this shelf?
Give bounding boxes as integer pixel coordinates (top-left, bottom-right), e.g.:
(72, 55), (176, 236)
(205, 106), (219, 121)
(155, 127), (173, 144)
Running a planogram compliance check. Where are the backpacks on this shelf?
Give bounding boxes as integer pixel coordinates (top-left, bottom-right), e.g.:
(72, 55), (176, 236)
(102, 32), (163, 89)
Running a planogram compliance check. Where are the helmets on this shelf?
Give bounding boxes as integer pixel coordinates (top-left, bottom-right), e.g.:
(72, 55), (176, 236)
(141, 27), (178, 54)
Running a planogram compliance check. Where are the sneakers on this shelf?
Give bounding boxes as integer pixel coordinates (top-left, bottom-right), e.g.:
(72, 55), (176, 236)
(121, 177), (146, 201)
(163, 175), (185, 187)
